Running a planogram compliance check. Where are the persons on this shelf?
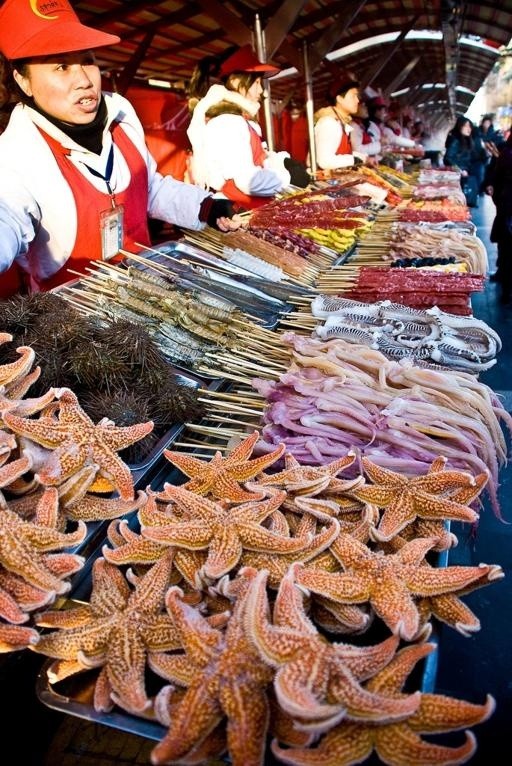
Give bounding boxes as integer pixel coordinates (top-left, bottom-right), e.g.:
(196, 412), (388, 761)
(110, 219), (117, 230)
(483, 132), (512, 283)
(183, 43), (311, 216)
(444, 117), (511, 208)
(306, 77), (431, 180)
(1, 0), (243, 293)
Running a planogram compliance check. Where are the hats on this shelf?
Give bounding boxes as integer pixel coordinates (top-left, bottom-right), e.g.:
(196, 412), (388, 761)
(220, 44), (279, 76)
(0, 0), (120, 59)
(366, 97), (384, 106)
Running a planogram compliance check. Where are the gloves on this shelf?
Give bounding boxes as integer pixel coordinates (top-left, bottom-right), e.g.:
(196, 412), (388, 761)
(284, 158), (310, 188)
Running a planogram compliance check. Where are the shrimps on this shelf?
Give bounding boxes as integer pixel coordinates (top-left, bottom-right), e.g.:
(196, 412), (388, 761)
(76, 268), (248, 370)
